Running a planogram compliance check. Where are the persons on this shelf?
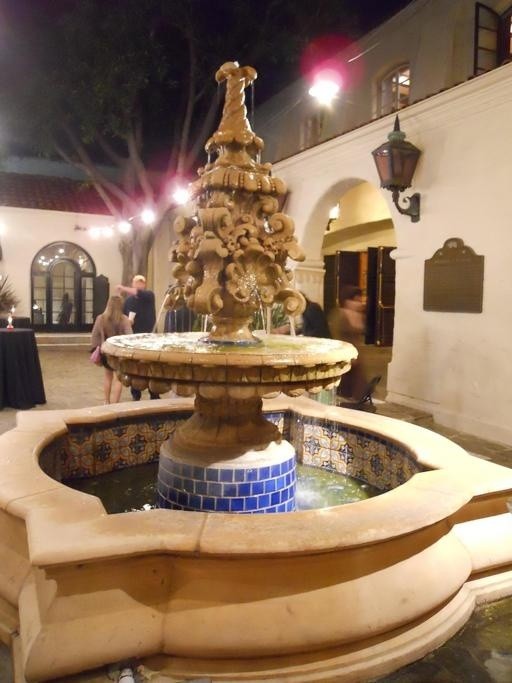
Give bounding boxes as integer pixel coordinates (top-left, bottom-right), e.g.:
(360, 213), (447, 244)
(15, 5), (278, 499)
(115, 274), (161, 401)
(270, 284), (370, 405)
(57, 291), (73, 325)
(32, 299), (42, 313)
(88, 295), (134, 406)
(163, 276), (198, 399)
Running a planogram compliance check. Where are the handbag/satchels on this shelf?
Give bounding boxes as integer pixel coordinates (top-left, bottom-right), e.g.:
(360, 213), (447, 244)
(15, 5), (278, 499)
(90, 346), (103, 368)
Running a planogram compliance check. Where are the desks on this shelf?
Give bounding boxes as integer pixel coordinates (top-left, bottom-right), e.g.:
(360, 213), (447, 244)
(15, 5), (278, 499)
(0, 315), (46, 412)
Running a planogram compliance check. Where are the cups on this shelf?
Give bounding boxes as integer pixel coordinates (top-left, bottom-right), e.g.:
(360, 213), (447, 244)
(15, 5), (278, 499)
(128, 312), (136, 321)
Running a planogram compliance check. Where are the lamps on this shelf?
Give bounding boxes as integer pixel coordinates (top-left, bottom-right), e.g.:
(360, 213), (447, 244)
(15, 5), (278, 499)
(370, 113), (422, 223)
